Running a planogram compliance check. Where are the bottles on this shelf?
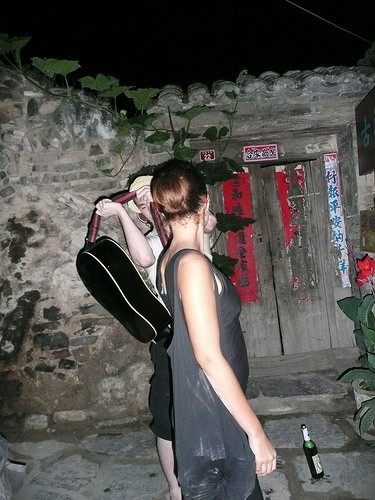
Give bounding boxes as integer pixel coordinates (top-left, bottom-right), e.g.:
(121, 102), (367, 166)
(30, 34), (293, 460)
(301, 423), (325, 480)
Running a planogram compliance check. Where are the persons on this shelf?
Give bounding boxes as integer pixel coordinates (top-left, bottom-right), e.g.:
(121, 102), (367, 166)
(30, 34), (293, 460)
(150, 158), (277, 500)
(96, 176), (218, 500)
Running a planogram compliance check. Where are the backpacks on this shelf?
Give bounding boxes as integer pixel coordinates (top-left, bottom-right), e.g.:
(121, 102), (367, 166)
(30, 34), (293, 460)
(77, 191), (172, 343)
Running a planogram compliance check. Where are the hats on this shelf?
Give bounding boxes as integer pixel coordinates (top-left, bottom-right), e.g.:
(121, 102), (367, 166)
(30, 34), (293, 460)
(128, 176), (153, 214)
